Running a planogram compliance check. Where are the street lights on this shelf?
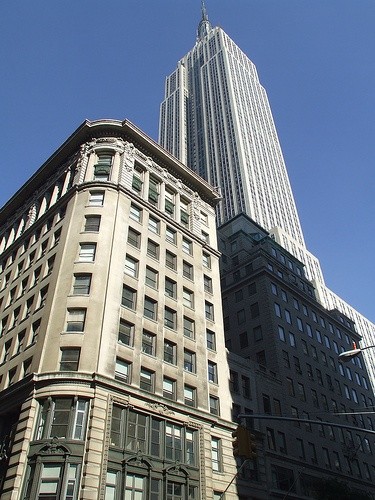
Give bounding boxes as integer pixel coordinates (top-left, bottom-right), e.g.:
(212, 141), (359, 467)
(339, 345), (375, 362)
